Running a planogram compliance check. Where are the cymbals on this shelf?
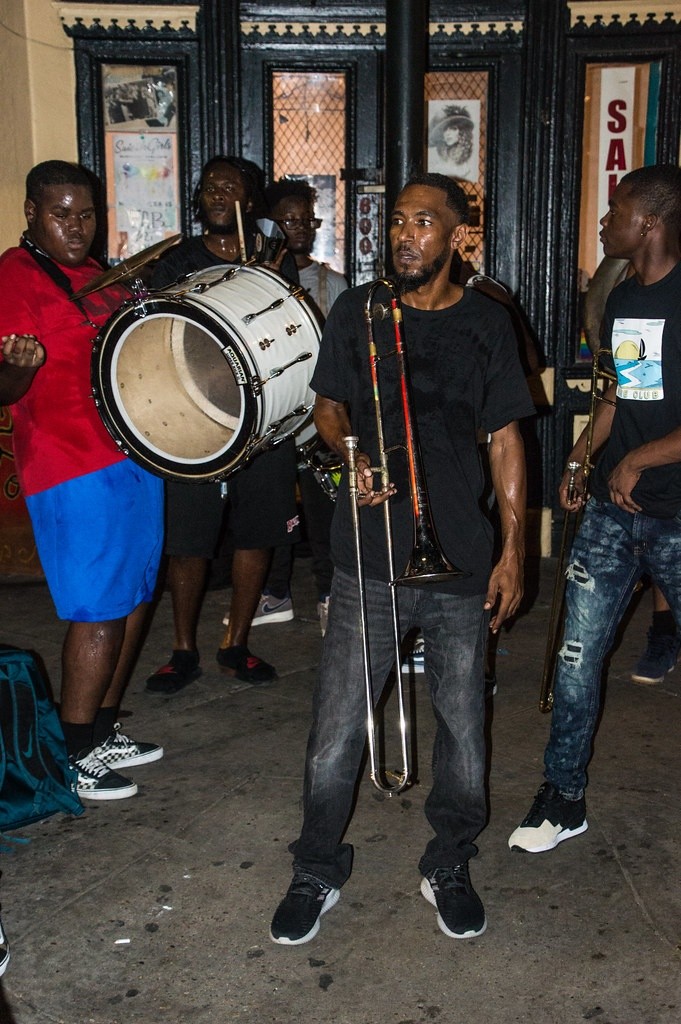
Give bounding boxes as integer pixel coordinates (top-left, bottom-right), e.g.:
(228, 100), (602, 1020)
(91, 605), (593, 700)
(66, 233), (186, 303)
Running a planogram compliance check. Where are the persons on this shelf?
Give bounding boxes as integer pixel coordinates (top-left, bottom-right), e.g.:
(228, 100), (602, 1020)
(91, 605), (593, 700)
(141, 158), (301, 692)
(629, 586), (680, 686)
(0, 160), (166, 801)
(507, 164), (681, 853)
(269, 174), (552, 948)
(221, 180), (348, 635)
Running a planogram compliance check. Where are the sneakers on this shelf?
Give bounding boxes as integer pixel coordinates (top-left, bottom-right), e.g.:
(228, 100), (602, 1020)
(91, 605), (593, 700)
(68, 746), (138, 800)
(89, 723), (164, 770)
(633, 626), (674, 685)
(508, 782), (589, 853)
(223, 592), (294, 626)
(391, 640), (425, 674)
(485, 674), (497, 701)
(270, 874), (340, 946)
(316, 596), (330, 638)
(420, 861), (487, 939)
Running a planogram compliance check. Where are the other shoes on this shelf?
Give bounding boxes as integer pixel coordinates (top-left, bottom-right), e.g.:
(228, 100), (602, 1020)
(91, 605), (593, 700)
(216, 644), (278, 687)
(146, 651), (199, 697)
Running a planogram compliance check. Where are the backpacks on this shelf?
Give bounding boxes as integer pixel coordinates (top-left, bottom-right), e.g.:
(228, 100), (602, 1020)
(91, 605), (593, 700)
(0, 645), (84, 831)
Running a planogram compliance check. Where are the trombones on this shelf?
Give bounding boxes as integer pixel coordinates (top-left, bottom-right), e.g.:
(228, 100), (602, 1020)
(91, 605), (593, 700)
(341, 278), (473, 798)
(538, 347), (616, 714)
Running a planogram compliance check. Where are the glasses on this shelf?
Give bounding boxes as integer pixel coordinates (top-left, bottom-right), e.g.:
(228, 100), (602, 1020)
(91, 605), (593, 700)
(274, 219), (323, 231)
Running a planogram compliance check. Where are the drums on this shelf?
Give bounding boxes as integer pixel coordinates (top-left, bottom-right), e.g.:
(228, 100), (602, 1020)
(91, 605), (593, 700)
(89, 263), (323, 486)
